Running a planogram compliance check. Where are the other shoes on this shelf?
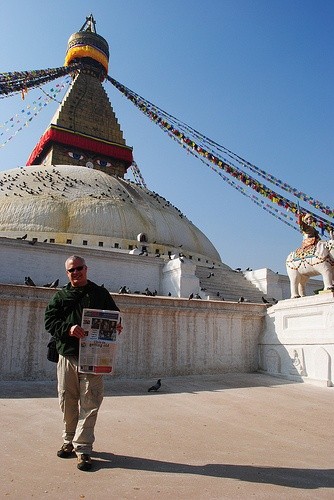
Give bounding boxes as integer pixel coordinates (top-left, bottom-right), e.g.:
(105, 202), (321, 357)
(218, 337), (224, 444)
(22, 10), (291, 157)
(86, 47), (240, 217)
(76, 453), (92, 471)
(57, 443), (74, 458)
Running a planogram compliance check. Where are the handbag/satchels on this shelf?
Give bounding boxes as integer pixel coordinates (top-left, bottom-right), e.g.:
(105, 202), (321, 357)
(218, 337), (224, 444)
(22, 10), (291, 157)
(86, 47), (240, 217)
(46, 335), (60, 363)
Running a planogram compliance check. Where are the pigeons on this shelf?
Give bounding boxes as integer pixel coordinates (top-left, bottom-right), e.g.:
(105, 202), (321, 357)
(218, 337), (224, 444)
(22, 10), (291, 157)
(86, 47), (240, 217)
(21, 234), (28, 240)
(134, 241), (279, 278)
(24, 276), (279, 304)
(0, 165), (187, 219)
(148, 379), (162, 391)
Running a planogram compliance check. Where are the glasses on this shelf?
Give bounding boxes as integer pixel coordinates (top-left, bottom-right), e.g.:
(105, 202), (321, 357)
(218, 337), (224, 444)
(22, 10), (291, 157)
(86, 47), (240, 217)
(66, 264), (87, 272)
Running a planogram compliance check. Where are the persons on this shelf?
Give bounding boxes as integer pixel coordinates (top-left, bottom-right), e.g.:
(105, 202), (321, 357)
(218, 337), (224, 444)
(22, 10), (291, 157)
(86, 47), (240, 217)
(44, 255), (123, 471)
(297, 211), (319, 247)
